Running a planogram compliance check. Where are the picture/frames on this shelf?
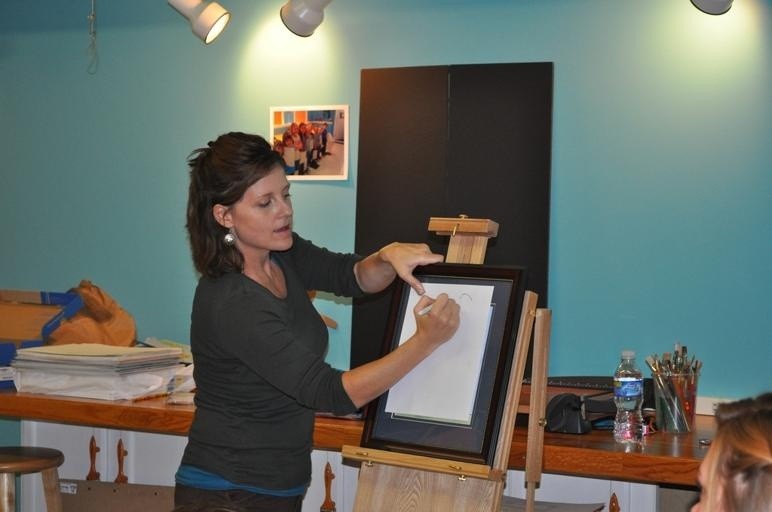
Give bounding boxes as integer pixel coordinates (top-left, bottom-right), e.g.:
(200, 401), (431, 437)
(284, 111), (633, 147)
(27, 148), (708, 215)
(268, 105), (350, 182)
(359, 261), (529, 468)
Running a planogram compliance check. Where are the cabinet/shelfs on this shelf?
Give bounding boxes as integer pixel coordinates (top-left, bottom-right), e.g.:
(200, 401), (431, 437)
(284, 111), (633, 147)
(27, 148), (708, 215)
(341, 214), (551, 511)
(0, 394), (725, 512)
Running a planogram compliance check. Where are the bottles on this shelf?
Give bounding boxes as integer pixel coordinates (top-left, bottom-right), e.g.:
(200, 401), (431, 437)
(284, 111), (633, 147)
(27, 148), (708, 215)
(612, 352), (645, 445)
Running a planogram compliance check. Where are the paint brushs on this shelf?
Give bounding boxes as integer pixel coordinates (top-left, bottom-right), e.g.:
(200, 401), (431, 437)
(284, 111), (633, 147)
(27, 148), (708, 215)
(646, 342), (702, 373)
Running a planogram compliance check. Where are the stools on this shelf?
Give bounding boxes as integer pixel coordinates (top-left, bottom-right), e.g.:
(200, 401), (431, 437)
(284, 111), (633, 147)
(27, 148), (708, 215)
(1, 444), (66, 511)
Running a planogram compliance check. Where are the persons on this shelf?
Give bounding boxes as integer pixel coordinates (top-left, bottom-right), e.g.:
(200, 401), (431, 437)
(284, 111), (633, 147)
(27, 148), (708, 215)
(274, 119), (333, 176)
(688, 392), (772, 512)
(174, 129), (461, 512)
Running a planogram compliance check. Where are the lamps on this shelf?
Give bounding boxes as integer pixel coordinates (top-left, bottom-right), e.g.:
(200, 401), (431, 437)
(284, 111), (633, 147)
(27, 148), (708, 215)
(167, 0), (330, 46)
(688, 1), (734, 16)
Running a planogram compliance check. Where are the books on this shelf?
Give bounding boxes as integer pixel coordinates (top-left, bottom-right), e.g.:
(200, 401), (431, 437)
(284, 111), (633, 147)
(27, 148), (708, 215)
(8, 339), (187, 400)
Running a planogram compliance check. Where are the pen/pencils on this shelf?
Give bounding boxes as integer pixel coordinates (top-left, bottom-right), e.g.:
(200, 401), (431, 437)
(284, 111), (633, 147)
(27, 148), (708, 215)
(418, 302), (436, 316)
